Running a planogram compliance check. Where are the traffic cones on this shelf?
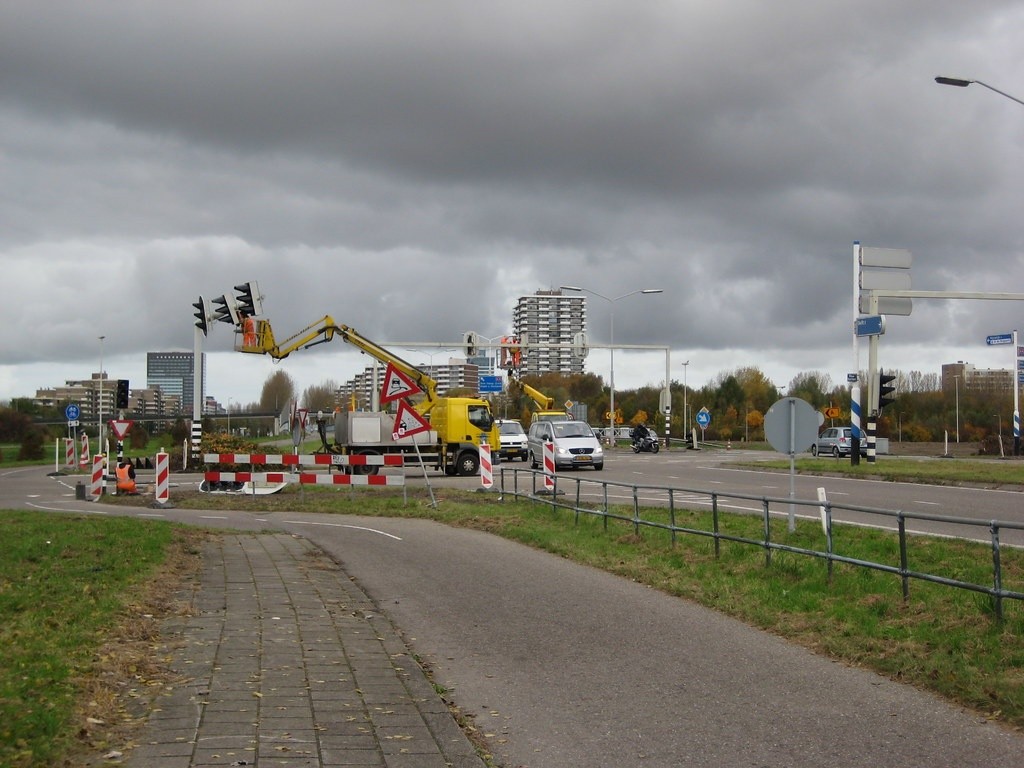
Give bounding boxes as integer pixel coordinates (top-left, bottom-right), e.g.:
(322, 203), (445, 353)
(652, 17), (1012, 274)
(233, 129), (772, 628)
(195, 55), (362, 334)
(727, 438), (731, 451)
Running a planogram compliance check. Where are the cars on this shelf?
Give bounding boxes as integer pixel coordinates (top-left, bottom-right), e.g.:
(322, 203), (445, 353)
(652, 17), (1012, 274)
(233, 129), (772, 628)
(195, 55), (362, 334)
(812, 426), (868, 458)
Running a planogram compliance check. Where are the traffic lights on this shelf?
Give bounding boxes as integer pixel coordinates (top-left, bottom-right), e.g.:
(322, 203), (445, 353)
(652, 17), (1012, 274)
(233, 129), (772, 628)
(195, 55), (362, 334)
(211, 292), (241, 326)
(234, 281), (263, 316)
(873, 367), (896, 412)
(197, 480), (247, 495)
(117, 378), (129, 410)
(192, 296), (208, 338)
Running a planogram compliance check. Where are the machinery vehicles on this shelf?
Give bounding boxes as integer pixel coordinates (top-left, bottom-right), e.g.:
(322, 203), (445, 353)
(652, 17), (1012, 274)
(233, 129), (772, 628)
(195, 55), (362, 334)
(233, 312), (502, 478)
(495, 348), (572, 424)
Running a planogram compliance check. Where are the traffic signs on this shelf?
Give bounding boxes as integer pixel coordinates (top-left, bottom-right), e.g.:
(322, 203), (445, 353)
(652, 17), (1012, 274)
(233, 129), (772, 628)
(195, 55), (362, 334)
(855, 315), (887, 337)
(823, 408), (840, 418)
(986, 334), (1013, 346)
(606, 412), (616, 420)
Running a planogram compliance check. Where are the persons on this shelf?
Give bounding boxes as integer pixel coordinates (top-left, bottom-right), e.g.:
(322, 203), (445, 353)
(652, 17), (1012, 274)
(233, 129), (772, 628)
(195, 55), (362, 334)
(575, 426), (580, 434)
(501, 335), (520, 366)
(240, 315), (256, 347)
(634, 422), (649, 448)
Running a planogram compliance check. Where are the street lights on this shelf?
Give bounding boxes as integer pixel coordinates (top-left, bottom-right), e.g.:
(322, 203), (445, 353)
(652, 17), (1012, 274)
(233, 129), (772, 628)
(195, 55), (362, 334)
(993, 415), (1001, 436)
(460, 332), (517, 401)
(96, 335), (106, 455)
(898, 412), (905, 442)
(406, 348), (456, 379)
(952, 375), (959, 444)
(681, 361), (690, 440)
(559, 286), (665, 447)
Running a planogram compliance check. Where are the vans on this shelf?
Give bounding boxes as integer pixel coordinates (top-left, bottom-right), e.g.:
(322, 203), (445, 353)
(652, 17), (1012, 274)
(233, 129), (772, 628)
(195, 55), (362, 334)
(528, 420), (604, 472)
(494, 419), (529, 462)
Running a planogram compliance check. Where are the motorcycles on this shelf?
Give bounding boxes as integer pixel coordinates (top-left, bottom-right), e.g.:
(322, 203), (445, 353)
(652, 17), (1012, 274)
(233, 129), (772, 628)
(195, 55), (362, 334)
(630, 429), (660, 454)
